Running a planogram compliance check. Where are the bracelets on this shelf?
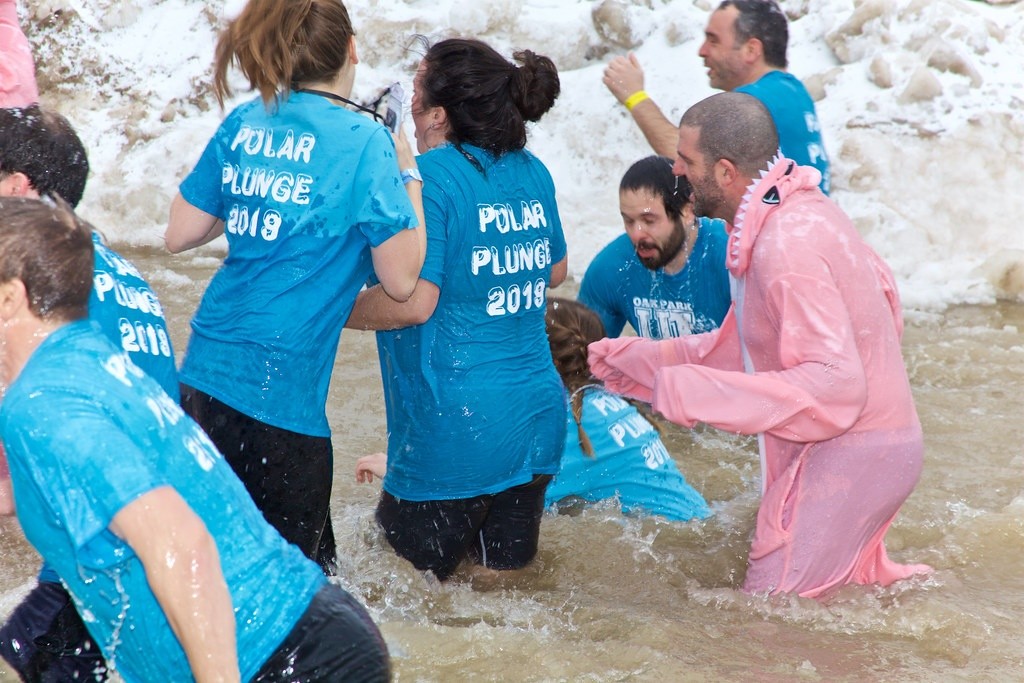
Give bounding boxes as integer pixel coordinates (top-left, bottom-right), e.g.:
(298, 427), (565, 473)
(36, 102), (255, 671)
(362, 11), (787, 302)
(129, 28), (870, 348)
(399, 169), (424, 187)
(624, 89), (647, 111)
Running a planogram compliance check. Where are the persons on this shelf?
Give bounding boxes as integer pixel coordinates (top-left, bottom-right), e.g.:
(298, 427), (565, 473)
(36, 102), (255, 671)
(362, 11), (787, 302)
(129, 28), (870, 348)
(2, 0), (44, 112)
(0, 104), (178, 682)
(357, 295), (706, 520)
(362, 37), (573, 581)
(162, 0), (427, 579)
(588, 91), (927, 607)
(575, 154), (733, 338)
(0, 192), (398, 682)
(603, 1), (831, 197)
